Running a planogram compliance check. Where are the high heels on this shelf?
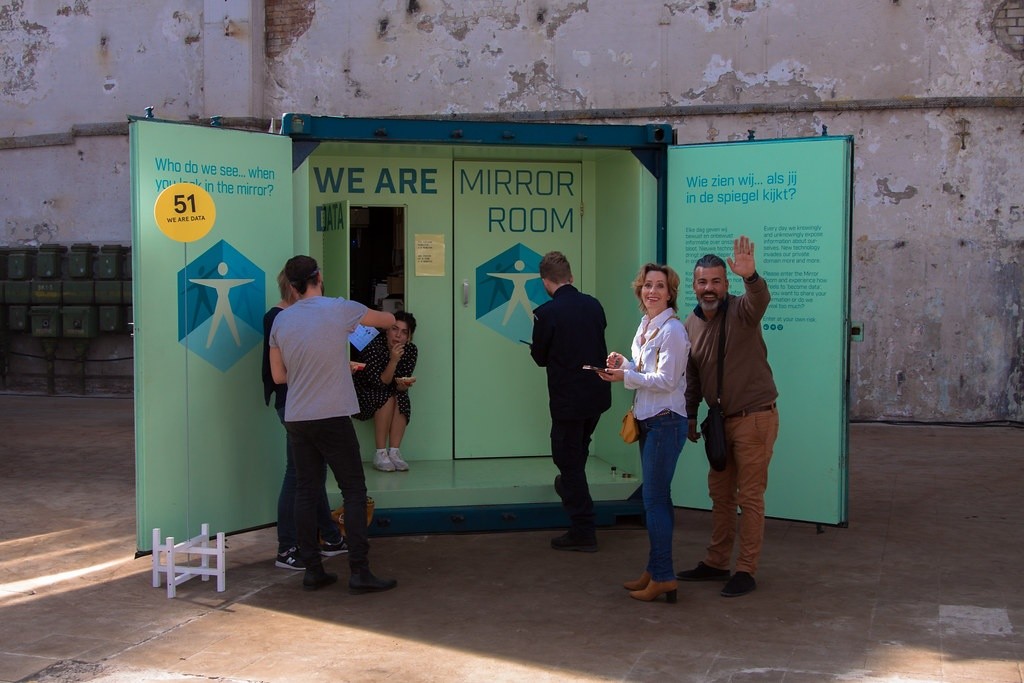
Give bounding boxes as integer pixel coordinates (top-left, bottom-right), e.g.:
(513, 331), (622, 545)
(630, 580), (678, 604)
(623, 570), (653, 591)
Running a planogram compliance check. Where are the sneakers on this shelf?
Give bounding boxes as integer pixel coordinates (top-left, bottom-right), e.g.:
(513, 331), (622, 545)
(674, 558), (730, 580)
(554, 475), (564, 503)
(275, 548), (307, 570)
(373, 450), (395, 471)
(388, 449), (409, 471)
(319, 538), (351, 556)
(720, 570), (756, 596)
(550, 534), (598, 552)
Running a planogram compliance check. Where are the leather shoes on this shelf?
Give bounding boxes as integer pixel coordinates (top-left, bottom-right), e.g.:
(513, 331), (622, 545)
(302, 571), (338, 590)
(347, 573), (397, 593)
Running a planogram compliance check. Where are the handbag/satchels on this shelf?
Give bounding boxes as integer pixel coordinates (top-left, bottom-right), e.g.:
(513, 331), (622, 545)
(700, 403), (729, 472)
(319, 497), (375, 542)
(621, 410), (639, 443)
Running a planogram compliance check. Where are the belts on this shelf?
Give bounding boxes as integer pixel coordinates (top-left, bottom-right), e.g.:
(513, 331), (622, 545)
(728, 403), (777, 418)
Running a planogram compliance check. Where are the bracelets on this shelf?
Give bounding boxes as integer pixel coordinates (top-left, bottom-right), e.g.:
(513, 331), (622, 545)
(746, 271), (756, 281)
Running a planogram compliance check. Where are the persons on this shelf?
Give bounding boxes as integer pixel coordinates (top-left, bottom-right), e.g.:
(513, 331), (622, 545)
(530, 251), (612, 553)
(261, 269), (347, 570)
(353, 312), (418, 472)
(595, 263), (691, 604)
(677, 235), (779, 597)
(269, 256), (398, 594)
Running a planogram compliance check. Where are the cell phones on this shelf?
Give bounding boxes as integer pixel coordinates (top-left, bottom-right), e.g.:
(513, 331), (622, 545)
(395, 377), (417, 382)
(582, 365), (612, 375)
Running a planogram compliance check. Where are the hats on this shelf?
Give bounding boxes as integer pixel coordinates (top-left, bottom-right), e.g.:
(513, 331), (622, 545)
(286, 256), (319, 285)
